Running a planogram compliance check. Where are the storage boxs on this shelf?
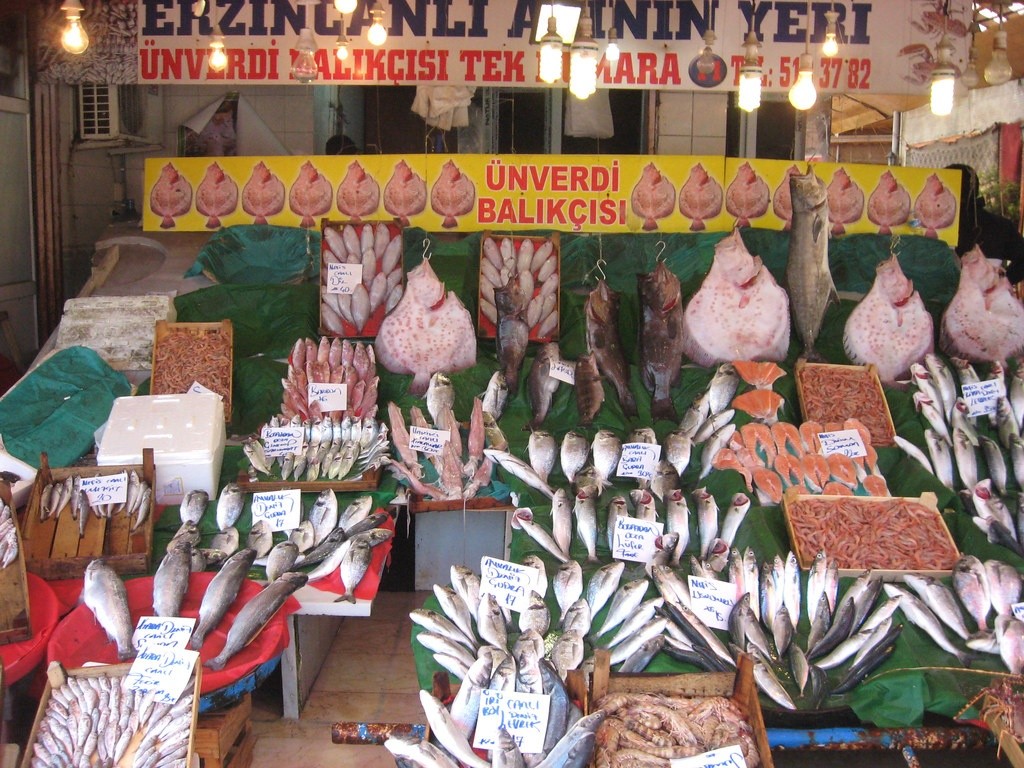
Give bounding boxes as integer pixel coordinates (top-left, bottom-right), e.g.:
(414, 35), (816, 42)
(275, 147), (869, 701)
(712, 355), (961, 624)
(97, 395), (227, 504)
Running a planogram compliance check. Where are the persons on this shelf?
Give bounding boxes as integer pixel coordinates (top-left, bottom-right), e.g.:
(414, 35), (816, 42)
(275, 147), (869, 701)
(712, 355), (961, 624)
(945, 164), (1023, 289)
(326, 134), (357, 154)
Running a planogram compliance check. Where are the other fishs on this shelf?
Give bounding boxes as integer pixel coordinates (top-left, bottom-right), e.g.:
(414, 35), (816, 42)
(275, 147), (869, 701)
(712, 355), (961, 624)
(243, 335), (392, 481)
(481, 236), (559, 338)
(31, 675), (194, 768)
(941, 242), (1024, 373)
(586, 260), (686, 425)
(38, 470), (152, 536)
(841, 251), (935, 389)
(684, 229), (790, 365)
(789, 165), (849, 356)
(492, 277), (562, 437)
(373, 260), (478, 398)
(385, 356), (1024, 768)
(322, 222), (406, 339)
(0, 499), (18, 571)
(80, 556), (133, 661)
(153, 481), (392, 669)
